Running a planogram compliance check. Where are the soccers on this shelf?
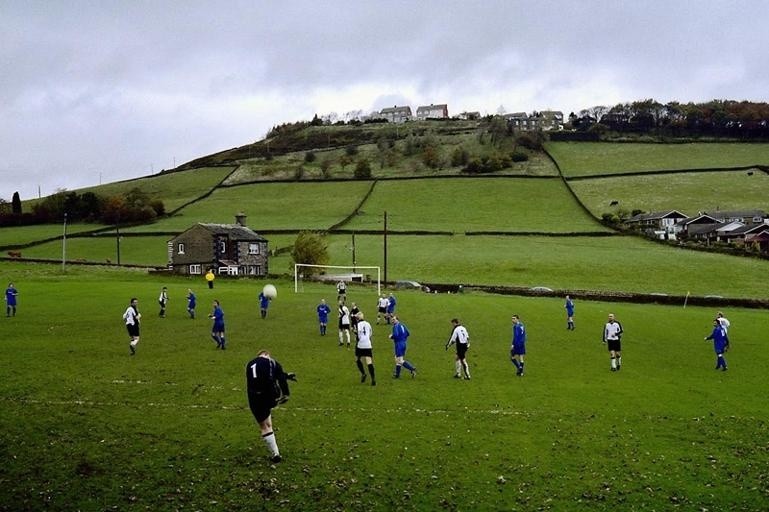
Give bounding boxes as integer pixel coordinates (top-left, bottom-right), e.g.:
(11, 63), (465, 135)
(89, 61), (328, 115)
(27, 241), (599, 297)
(263, 284), (278, 298)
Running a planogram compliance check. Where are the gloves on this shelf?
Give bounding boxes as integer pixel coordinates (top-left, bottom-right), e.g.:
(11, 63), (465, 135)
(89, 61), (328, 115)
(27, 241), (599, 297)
(288, 373), (297, 383)
(275, 396), (289, 405)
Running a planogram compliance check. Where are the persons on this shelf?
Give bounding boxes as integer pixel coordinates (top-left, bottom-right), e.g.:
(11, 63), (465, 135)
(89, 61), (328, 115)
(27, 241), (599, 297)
(185, 288), (196, 318)
(4, 282), (20, 318)
(257, 292), (272, 318)
(389, 315), (416, 378)
(717, 313), (731, 335)
(244, 352), (296, 464)
(445, 319), (471, 381)
(564, 295), (575, 330)
(335, 279), (397, 349)
(204, 269), (215, 289)
(208, 300), (226, 351)
(157, 287), (168, 319)
(705, 318), (731, 372)
(123, 297), (142, 355)
(509, 315), (526, 378)
(602, 313), (625, 371)
(315, 299), (331, 336)
(354, 313), (376, 386)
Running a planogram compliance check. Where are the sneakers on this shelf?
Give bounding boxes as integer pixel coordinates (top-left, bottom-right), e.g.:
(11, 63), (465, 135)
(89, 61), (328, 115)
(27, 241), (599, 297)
(454, 367), (524, 381)
(261, 315), (265, 319)
(567, 326), (575, 331)
(5, 312), (16, 318)
(611, 365), (620, 371)
(271, 455), (281, 464)
(130, 314), (226, 356)
(320, 321), (416, 386)
(715, 365), (728, 371)
